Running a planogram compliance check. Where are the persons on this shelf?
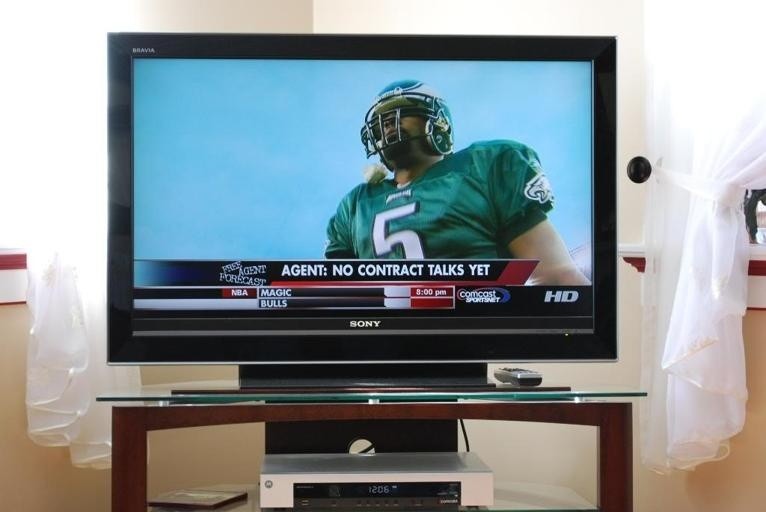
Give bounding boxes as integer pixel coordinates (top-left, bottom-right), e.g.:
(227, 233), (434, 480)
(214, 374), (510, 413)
(325, 78), (593, 286)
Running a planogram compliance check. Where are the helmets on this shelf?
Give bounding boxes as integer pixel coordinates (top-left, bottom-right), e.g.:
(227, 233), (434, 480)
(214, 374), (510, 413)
(360, 78), (455, 159)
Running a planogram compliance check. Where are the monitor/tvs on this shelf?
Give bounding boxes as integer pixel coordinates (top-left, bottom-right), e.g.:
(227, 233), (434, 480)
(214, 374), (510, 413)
(106, 30), (619, 392)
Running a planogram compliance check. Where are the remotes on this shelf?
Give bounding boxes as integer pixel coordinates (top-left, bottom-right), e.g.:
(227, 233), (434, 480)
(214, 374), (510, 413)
(495, 366), (543, 387)
